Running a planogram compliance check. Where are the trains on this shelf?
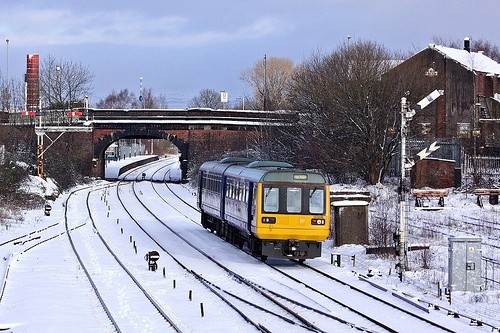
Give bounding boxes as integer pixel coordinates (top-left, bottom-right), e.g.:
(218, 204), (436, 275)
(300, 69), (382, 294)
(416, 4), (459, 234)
(196, 155), (331, 264)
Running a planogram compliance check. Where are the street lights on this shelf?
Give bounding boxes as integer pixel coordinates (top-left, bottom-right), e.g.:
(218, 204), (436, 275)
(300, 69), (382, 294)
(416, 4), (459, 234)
(263, 53), (267, 110)
(346, 35), (351, 49)
(5, 38), (10, 85)
